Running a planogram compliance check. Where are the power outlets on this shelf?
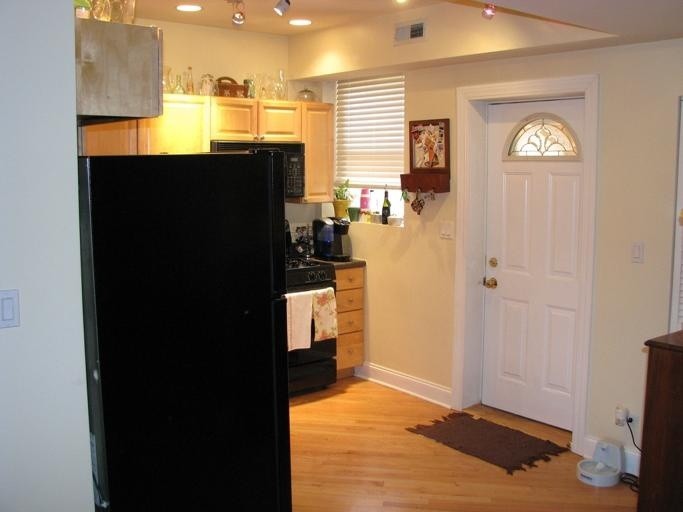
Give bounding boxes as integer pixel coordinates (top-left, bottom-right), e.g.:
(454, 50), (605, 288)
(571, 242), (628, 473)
(615, 406), (629, 427)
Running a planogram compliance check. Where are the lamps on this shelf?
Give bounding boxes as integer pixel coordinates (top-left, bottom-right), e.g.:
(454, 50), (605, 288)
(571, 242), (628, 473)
(483, 4), (497, 18)
(273, 0), (290, 17)
(227, 1), (246, 25)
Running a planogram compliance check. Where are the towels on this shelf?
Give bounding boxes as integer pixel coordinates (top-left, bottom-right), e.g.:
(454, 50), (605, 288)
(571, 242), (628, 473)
(284, 291), (312, 352)
(311, 287), (338, 341)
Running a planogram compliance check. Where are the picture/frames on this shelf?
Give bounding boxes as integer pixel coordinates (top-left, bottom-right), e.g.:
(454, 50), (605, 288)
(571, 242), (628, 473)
(409, 118), (450, 174)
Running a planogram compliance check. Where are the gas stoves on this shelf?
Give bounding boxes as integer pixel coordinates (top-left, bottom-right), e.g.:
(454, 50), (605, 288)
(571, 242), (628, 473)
(285, 244), (330, 287)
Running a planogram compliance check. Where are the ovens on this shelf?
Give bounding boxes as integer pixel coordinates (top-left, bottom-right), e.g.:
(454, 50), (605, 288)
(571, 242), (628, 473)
(286, 281), (337, 394)
(209, 140), (304, 198)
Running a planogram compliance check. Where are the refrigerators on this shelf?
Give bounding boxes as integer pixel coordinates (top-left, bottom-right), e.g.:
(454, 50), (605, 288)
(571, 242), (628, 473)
(77, 148), (292, 512)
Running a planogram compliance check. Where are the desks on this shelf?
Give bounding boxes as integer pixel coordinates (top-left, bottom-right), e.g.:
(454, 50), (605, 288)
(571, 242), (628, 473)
(637, 330), (683, 512)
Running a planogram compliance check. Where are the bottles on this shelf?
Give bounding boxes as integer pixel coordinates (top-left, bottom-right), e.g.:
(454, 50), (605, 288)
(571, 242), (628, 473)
(382, 191), (391, 225)
(186, 66), (194, 95)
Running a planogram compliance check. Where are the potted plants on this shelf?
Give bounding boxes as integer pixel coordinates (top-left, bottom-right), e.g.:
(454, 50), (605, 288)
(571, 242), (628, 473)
(332, 179), (352, 219)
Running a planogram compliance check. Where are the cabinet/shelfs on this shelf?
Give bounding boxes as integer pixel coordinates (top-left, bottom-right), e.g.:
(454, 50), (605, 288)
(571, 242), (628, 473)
(335, 261), (366, 380)
(78, 92), (210, 155)
(285, 102), (336, 204)
(211, 95), (301, 143)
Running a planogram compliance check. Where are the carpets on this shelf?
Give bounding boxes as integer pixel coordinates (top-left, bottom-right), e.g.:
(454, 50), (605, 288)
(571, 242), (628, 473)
(404, 411), (569, 477)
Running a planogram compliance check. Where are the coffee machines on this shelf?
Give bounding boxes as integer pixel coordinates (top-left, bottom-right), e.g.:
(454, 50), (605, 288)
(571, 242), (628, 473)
(312, 216), (353, 262)
